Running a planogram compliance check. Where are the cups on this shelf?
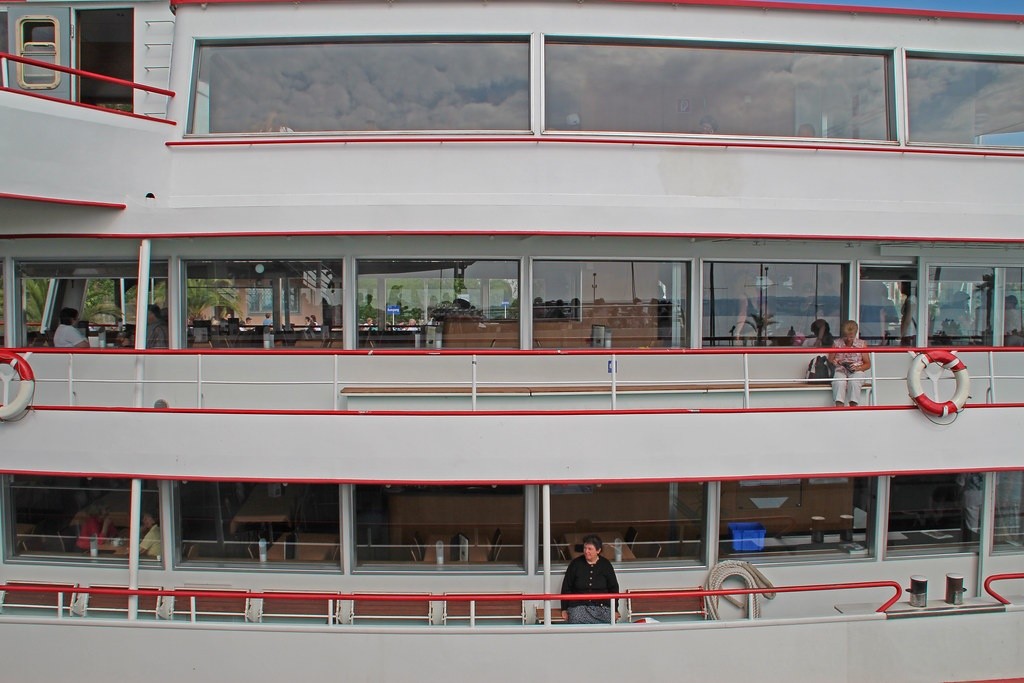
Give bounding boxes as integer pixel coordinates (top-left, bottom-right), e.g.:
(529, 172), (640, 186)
(111, 538), (123, 547)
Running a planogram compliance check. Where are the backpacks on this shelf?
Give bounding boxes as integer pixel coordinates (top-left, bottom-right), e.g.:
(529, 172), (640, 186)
(806, 355), (835, 384)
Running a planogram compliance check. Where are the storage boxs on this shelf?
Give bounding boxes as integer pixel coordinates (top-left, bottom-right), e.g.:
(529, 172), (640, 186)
(727, 522), (765, 552)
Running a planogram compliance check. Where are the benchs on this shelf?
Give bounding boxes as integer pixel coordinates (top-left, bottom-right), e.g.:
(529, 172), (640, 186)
(341, 376), (874, 406)
(531, 606), (621, 624)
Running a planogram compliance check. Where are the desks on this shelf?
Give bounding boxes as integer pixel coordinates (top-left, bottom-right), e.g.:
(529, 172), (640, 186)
(229, 482), (310, 543)
(423, 533), (491, 562)
(564, 530), (637, 562)
(70, 479), (158, 530)
(267, 532), (339, 561)
(82, 528), (146, 558)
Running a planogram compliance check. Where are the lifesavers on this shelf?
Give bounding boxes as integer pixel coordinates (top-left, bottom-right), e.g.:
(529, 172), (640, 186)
(907, 350), (969, 416)
(0, 350), (36, 421)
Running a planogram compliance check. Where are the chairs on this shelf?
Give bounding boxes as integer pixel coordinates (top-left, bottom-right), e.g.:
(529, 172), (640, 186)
(45, 328), (386, 348)
(406, 531), (425, 562)
(551, 533), (569, 560)
(186, 544), (200, 558)
(487, 528), (504, 561)
(58, 525), (80, 553)
(647, 540), (662, 559)
(623, 527), (638, 550)
(247, 529), (273, 559)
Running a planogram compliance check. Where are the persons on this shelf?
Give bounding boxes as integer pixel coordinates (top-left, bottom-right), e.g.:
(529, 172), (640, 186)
(803, 319), (834, 347)
(829, 320), (871, 407)
(561, 535), (621, 624)
(30, 296), (672, 348)
(75, 500), (120, 553)
(734, 275), (1024, 346)
(139, 514), (162, 560)
(933, 475), (982, 552)
